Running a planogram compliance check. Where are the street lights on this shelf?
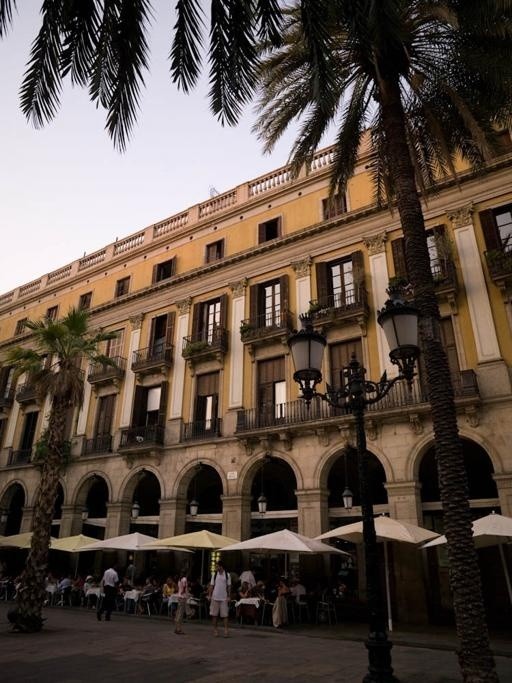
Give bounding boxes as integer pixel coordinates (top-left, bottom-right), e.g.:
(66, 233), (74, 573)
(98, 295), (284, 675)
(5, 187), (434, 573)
(284, 270), (428, 683)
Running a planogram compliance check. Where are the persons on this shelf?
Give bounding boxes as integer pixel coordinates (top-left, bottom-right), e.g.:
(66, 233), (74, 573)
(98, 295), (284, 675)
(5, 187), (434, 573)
(191, 576), (206, 599)
(120, 571), (192, 616)
(96, 563), (119, 621)
(127, 560), (137, 586)
(231, 566), (307, 620)
(44, 572), (98, 610)
(207, 561), (231, 638)
(326, 576), (352, 623)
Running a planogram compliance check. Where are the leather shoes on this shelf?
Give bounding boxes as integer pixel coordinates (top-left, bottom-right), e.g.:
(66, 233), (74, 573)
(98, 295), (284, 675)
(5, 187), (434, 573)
(97, 612), (111, 620)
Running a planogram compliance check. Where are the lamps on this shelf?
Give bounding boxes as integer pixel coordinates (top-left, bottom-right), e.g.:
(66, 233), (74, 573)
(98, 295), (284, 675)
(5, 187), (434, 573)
(131, 501), (140, 520)
(342, 449), (353, 509)
(80, 507), (88, 521)
(0, 509), (9, 525)
(189, 467), (200, 516)
(256, 461), (267, 513)
